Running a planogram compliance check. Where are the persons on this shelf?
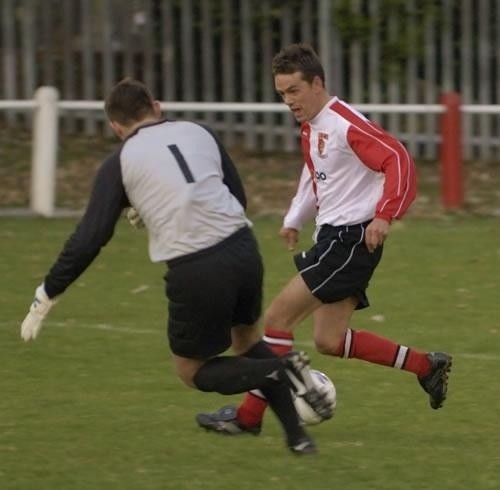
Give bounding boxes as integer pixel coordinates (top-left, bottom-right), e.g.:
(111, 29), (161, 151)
(20, 77), (336, 457)
(194, 43), (451, 434)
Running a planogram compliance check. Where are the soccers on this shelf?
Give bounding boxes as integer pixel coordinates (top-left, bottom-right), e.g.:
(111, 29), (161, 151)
(289, 370), (336, 427)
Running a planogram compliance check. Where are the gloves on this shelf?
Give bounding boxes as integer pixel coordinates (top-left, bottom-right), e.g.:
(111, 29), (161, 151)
(20, 281), (63, 343)
(127, 207), (146, 231)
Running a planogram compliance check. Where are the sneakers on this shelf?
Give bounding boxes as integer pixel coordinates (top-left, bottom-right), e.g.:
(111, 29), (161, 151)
(194, 403), (264, 437)
(286, 428), (318, 455)
(417, 350), (454, 411)
(277, 348), (335, 421)
